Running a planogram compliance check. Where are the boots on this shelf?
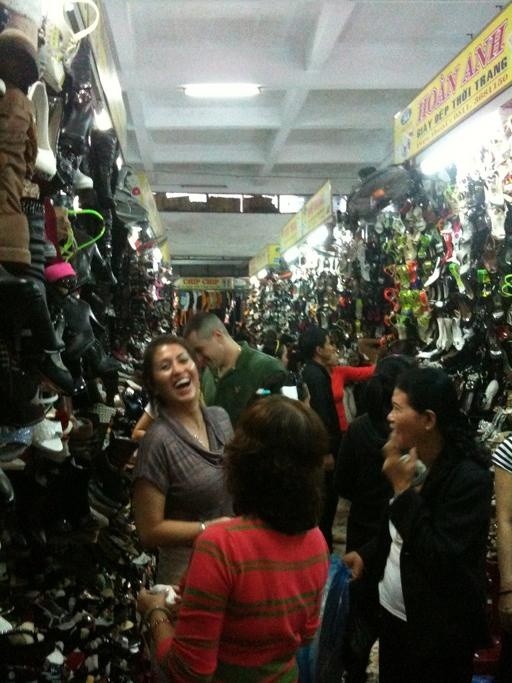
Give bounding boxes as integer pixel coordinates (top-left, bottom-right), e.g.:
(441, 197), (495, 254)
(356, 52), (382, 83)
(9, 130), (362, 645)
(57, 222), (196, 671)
(1, 2), (172, 683)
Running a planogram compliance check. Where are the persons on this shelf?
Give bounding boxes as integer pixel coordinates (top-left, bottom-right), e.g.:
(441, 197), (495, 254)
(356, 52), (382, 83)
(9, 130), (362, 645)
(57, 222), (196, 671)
(126, 311), (512, 683)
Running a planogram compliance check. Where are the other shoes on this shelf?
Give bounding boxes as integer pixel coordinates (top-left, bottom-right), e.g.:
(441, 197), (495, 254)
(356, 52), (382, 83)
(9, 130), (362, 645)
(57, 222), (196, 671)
(374, 147), (511, 450)
